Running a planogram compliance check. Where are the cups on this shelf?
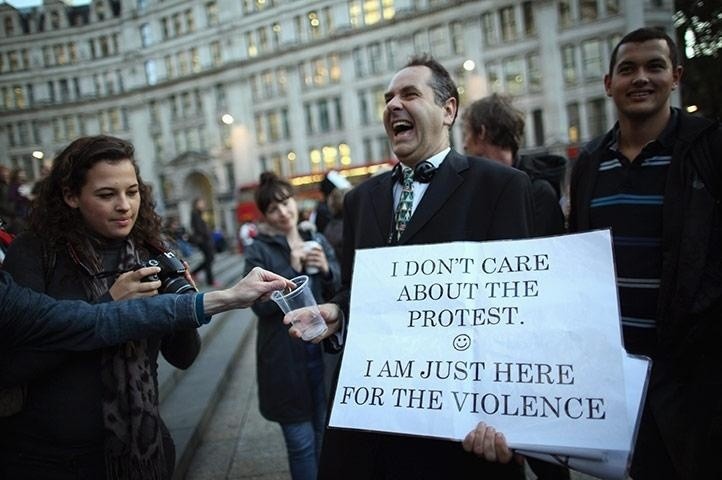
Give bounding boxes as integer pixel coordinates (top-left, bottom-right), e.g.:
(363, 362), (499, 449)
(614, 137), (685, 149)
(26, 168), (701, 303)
(270, 274), (327, 342)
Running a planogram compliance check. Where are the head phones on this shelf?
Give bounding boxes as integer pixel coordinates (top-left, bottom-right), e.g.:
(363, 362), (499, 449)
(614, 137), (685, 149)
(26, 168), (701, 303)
(391, 160), (437, 185)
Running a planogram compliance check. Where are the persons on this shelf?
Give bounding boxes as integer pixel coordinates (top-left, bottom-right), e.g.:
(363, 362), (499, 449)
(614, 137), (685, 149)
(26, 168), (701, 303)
(0, 27), (722, 480)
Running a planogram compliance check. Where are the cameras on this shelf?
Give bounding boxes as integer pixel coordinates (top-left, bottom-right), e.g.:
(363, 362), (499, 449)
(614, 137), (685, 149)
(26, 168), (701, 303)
(133, 252), (196, 294)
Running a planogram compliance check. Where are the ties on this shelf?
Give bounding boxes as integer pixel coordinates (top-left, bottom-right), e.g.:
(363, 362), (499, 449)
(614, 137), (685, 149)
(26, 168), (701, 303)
(394, 167), (415, 241)
